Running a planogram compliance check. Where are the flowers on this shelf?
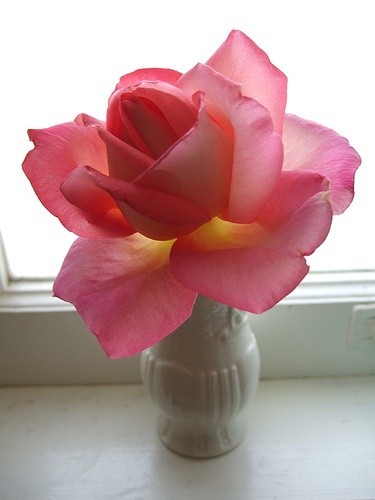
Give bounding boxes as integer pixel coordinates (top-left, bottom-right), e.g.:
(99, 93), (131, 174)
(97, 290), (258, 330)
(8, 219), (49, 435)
(22, 30), (360, 362)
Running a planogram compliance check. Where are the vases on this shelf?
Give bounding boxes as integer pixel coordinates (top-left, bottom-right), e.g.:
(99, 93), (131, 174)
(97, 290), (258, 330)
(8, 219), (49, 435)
(140, 292), (260, 457)
